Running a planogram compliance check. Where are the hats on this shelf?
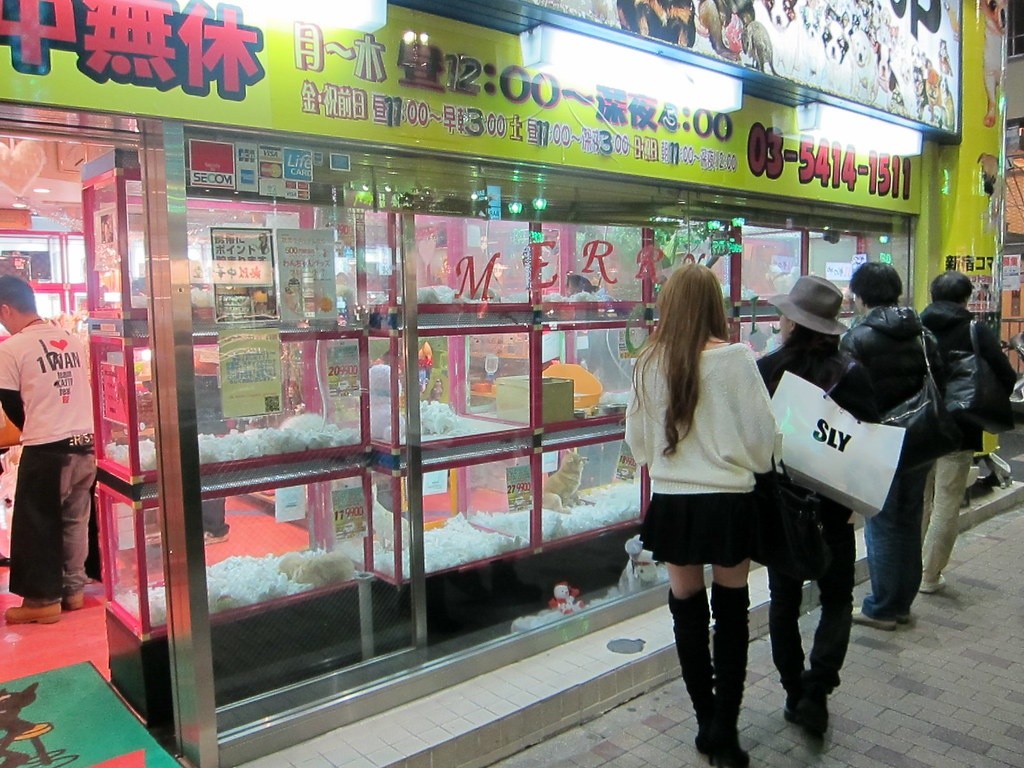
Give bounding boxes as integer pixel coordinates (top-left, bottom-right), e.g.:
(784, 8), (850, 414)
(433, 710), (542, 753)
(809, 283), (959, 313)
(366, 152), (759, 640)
(768, 275), (849, 336)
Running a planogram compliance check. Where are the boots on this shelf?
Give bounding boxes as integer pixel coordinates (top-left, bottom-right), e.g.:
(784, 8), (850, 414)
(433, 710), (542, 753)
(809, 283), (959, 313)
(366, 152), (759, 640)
(669, 586), (719, 753)
(712, 581), (749, 766)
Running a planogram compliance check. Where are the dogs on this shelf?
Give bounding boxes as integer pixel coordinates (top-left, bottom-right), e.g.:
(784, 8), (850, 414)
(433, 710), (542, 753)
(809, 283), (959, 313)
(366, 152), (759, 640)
(544, 448), (590, 515)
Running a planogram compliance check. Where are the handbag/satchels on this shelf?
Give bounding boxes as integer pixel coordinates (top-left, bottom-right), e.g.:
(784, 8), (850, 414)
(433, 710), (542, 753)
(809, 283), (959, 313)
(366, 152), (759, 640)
(877, 368), (965, 472)
(947, 351), (1015, 434)
(769, 370), (906, 519)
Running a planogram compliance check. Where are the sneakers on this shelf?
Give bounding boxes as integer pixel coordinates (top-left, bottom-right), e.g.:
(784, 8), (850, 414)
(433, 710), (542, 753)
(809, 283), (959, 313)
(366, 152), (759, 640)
(5, 596), (61, 624)
(852, 606), (912, 631)
(919, 573), (945, 594)
(62, 585), (84, 610)
(203, 523), (229, 545)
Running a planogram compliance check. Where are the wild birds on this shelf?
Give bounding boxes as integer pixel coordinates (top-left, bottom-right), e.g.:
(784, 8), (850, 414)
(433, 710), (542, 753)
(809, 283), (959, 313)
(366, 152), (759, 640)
(425, 379), (443, 404)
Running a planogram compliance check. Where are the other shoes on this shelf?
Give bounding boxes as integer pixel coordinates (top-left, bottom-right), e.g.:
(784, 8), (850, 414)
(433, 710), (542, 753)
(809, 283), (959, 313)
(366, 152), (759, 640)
(785, 688), (804, 722)
(800, 685), (828, 732)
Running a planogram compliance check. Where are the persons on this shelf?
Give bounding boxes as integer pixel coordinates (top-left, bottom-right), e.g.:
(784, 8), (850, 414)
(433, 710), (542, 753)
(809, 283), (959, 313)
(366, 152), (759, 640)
(0, 274), (96, 626)
(625, 268), (782, 768)
(920, 270), (1017, 594)
(756, 277), (883, 730)
(840, 262), (945, 629)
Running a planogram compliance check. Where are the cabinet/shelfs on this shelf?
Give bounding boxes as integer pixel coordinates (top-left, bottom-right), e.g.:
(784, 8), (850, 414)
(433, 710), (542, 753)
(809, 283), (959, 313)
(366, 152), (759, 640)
(81, 148), (915, 742)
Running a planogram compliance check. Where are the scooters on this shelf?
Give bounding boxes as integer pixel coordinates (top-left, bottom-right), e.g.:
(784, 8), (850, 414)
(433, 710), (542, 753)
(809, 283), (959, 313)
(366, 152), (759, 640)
(1002, 333), (1024, 416)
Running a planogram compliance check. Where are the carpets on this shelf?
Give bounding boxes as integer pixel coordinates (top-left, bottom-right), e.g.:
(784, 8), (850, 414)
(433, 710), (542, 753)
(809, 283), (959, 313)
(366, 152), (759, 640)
(1, 662), (186, 767)
